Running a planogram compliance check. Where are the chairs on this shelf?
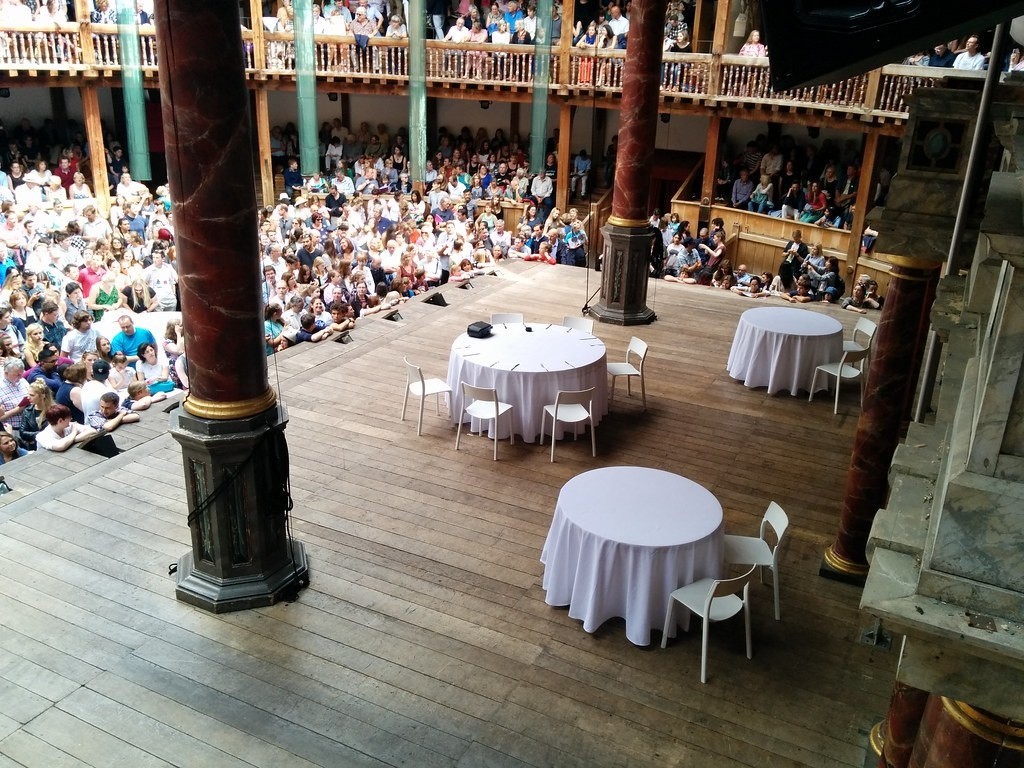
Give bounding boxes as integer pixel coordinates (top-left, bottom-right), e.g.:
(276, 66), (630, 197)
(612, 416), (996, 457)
(490, 313), (524, 326)
(563, 316), (594, 336)
(455, 380), (513, 461)
(539, 386), (596, 463)
(661, 562), (757, 682)
(809, 347), (869, 414)
(607, 336), (648, 412)
(723, 501), (788, 616)
(401, 356), (454, 436)
(842, 316), (876, 372)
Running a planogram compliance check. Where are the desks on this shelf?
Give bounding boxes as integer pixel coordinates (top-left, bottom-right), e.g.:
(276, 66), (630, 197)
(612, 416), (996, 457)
(725, 306), (842, 397)
(445, 324), (607, 443)
(538, 466), (722, 647)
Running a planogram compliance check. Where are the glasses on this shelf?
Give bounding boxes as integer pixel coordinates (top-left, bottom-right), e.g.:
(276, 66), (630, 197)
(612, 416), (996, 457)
(316, 218), (323, 221)
(25, 269), (34, 274)
(45, 359), (57, 363)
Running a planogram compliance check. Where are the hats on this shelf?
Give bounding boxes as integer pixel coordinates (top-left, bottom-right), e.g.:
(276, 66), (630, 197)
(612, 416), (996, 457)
(92, 360), (109, 381)
(822, 287), (838, 299)
(294, 196), (308, 207)
(278, 193), (291, 201)
(22, 170), (45, 186)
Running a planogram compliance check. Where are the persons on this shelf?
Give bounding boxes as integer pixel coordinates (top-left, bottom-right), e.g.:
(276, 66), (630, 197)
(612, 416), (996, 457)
(0, 431), (27, 467)
(0, 119), (193, 391)
(897, 27), (1023, 91)
(645, 121), (894, 316)
(35, 405), (98, 452)
(240, 1), (773, 95)
(252, 114), (618, 358)
(0, 357), (31, 447)
(1, 1), (158, 65)
(121, 381), (168, 411)
(86, 392), (140, 433)
(18, 377), (59, 451)
